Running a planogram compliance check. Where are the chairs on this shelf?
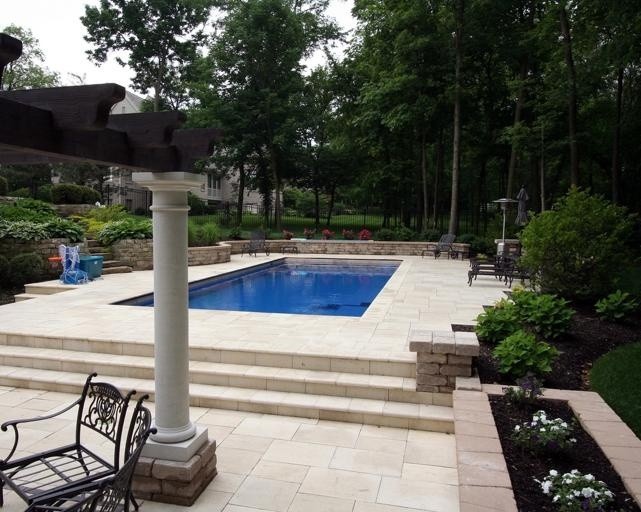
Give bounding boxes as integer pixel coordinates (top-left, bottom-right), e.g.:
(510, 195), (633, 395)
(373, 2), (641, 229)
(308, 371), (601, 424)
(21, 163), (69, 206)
(421, 232), (464, 260)
(465, 251), (533, 288)
(29, 394), (158, 507)
(0, 373), (136, 508)
(239, 230), (299, 257)
